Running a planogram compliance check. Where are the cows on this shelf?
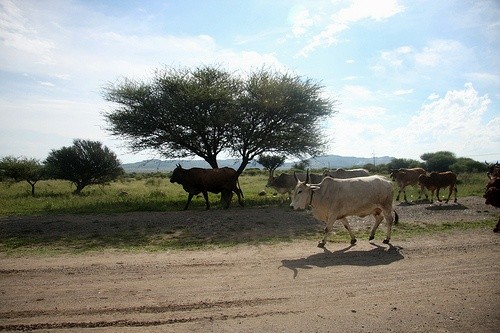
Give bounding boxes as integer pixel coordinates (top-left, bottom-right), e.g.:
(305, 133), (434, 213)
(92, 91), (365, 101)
(388, 168), (429, 201)
(482, 160), (500, 233)
(263, 164), (400, 248)
(418, 171), (458, 204)
(169, 163), (244, 210)
